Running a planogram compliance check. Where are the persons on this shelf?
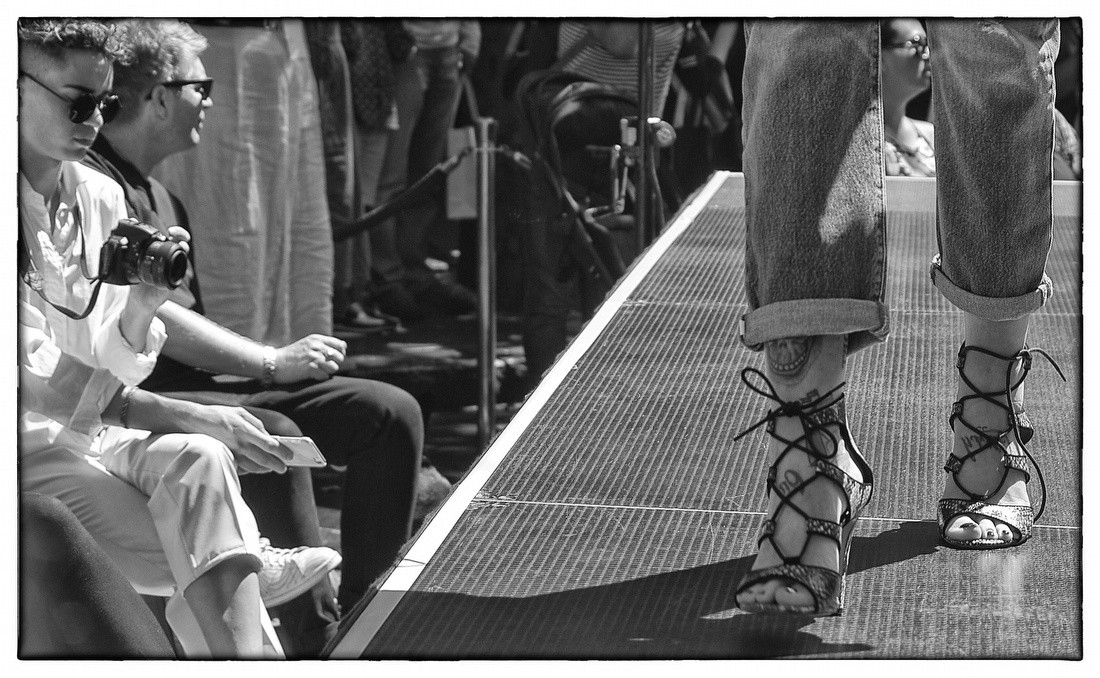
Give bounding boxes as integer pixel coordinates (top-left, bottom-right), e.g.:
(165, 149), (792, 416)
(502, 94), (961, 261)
(172, 19), (746, 389)
(729, 19), (1062, 618)
(94, 18), (424, 656)
(878, 19), (937, 178)
(1051, 107), (1081, 181)
(18, 18), (341, 659)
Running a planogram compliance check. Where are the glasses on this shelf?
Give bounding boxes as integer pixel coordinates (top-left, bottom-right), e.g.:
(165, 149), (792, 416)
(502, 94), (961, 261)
(145, 76), (216, 103)
(18, 68), (123, 125)
(879, 36), (932, 60)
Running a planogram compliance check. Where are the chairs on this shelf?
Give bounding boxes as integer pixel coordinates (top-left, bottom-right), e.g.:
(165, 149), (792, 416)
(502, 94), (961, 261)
(521, 21), (685, 266)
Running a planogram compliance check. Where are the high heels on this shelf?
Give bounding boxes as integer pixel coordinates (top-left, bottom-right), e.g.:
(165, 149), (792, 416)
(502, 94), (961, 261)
(937, 341), (1067, 549)
(732, 366), (873, 620)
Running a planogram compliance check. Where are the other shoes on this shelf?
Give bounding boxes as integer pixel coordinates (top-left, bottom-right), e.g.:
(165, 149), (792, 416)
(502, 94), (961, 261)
(330, 306), (405, 340)
(382, 278), (439, 319)
(423, 275), (477, 315)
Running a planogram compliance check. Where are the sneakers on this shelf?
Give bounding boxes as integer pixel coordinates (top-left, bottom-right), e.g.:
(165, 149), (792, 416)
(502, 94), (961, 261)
(250, 537), (343, 608)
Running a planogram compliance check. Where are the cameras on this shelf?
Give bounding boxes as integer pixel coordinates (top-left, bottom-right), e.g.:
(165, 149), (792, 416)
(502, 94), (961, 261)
(98, 217), (187, 291)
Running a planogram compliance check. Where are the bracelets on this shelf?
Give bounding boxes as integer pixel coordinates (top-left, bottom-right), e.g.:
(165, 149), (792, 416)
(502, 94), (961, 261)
(259, 345), (277, 389)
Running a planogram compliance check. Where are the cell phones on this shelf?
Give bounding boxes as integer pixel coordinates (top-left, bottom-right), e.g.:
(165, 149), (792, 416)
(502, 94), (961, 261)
(268, 435), (327, 469)
